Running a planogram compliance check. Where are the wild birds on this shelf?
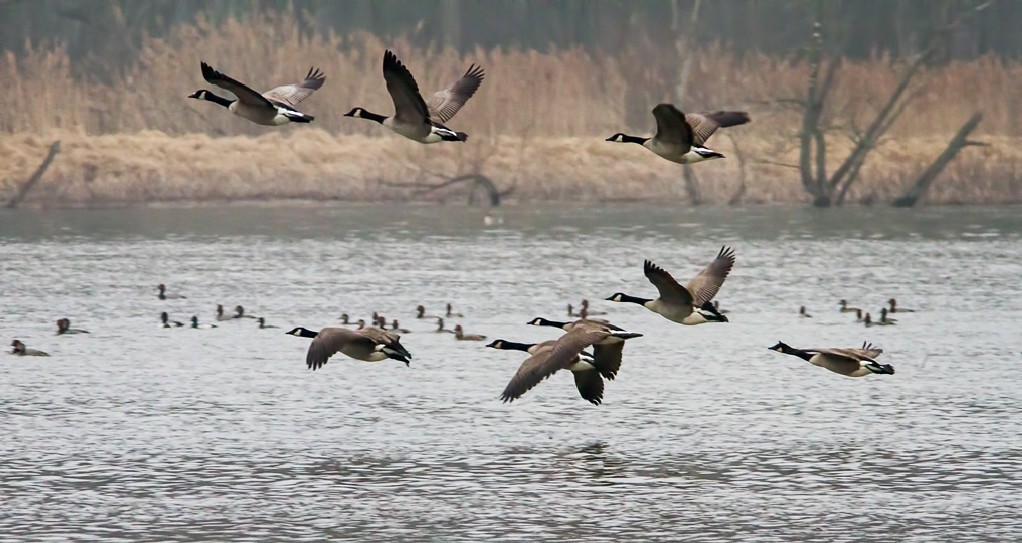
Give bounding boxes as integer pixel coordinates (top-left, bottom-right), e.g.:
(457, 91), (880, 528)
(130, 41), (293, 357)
(187, 61), (326, 126)
(526, 317), (643, 381)
(191, 304), (279, 330)
(767, 342), (894, 377)
(800, 298), (912, 326)
(286, 326), (412, 371)
(485, 339), (605, 405)
(436, 319), (453, 333)
(159, 312), (182, 328)
(339, 314), (357, 324)
(343, 49), (485, 145)
(415, 305), (439, 317)
(604, 244), (736, 325)
(567, 300), (607, 317)
(358, 311), (409, 333)
(605, 103), (751, 165)
(157, 284), (185, 299)
(455, 326), (486, 341)
(10, 319), (90, 356)
(446, 304), (462, 318)
